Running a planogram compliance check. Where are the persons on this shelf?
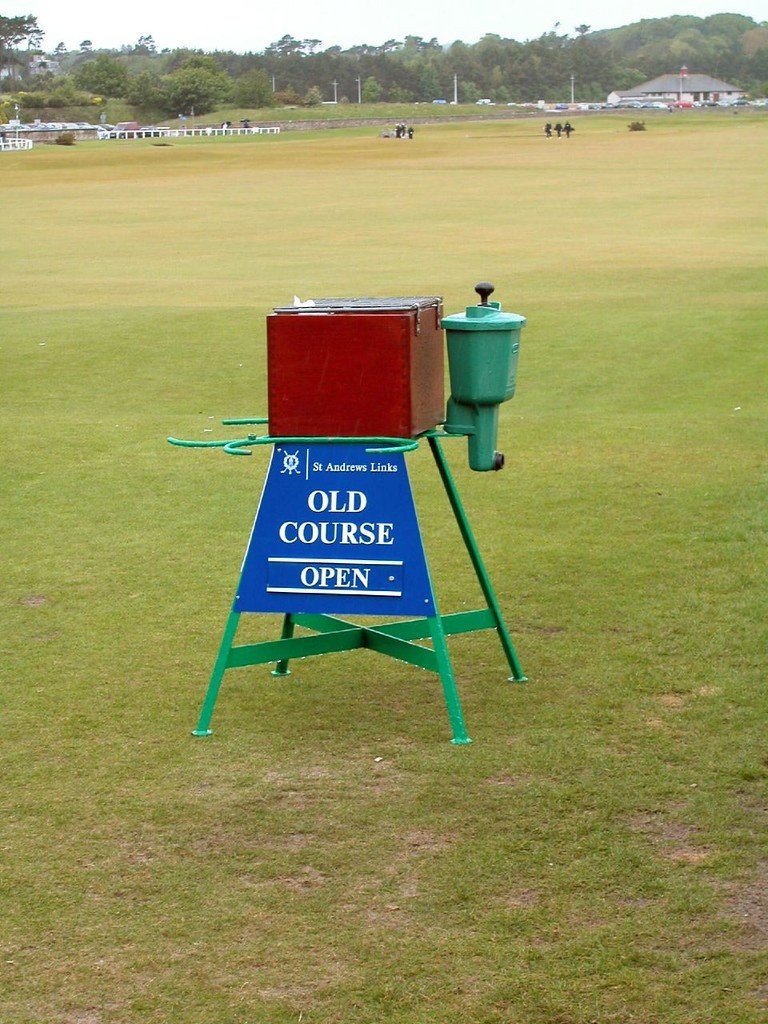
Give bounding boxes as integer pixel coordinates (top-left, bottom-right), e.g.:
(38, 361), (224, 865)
(546, 121), (552, 137)
(556, 123), (562, 137)
(408, 127), (414, 139)
(564, 121), (571, 138)
(395, 123), (407, 138)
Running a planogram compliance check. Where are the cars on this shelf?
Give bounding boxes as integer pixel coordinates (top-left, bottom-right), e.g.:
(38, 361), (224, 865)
(0, 122), (164, 139)
(555, 100), (750, 110)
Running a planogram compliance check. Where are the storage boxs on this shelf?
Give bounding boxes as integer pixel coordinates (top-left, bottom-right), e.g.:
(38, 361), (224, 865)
(264, 291), (448, 439)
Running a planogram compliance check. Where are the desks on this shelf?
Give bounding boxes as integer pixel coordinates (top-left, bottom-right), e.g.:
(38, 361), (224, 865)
(165, 418), (528, 747)
